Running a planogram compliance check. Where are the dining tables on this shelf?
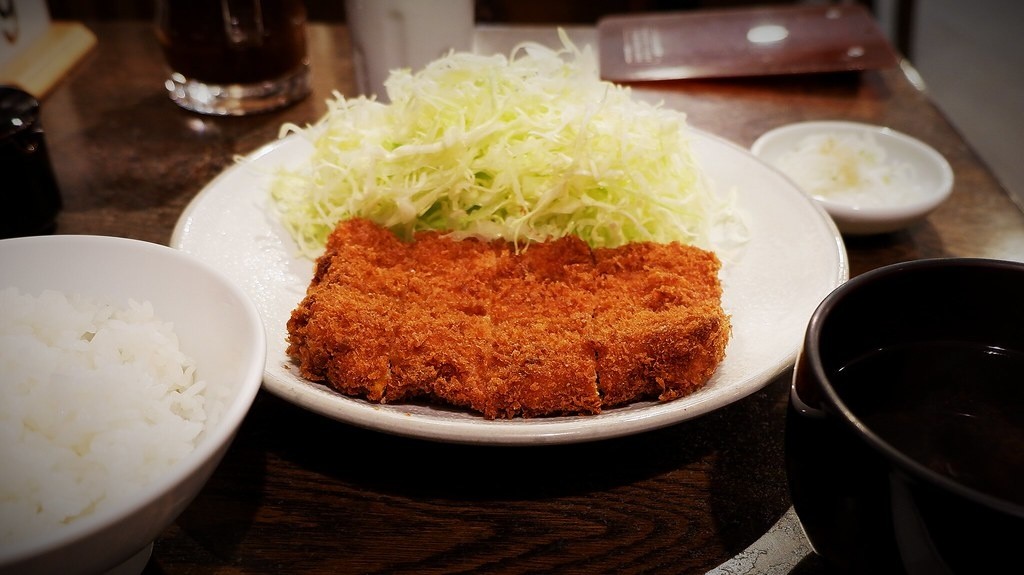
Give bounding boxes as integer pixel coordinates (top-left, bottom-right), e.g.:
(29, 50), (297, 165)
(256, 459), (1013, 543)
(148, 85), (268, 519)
(0, 17), (1024, 574)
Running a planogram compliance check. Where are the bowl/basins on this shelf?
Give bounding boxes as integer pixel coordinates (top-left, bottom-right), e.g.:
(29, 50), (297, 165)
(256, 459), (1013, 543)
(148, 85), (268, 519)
(0, 236), (268, 575)
(780, 259), (1024, 575)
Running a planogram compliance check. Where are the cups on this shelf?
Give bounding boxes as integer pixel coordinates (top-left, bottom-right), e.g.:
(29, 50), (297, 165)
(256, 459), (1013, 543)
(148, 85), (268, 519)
(151, 0), (313, 116)
(0, 85), (62, 230)
(346, 0), (472, 100)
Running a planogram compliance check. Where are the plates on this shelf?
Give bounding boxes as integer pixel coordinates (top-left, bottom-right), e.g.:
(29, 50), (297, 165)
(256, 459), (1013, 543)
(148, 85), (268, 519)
(746, 120), (952, 233)
(171, 120), (849, 444)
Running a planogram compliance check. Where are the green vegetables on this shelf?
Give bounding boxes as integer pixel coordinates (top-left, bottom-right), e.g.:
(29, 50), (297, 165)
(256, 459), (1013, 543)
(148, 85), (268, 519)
(254, 27), (696, 261)
